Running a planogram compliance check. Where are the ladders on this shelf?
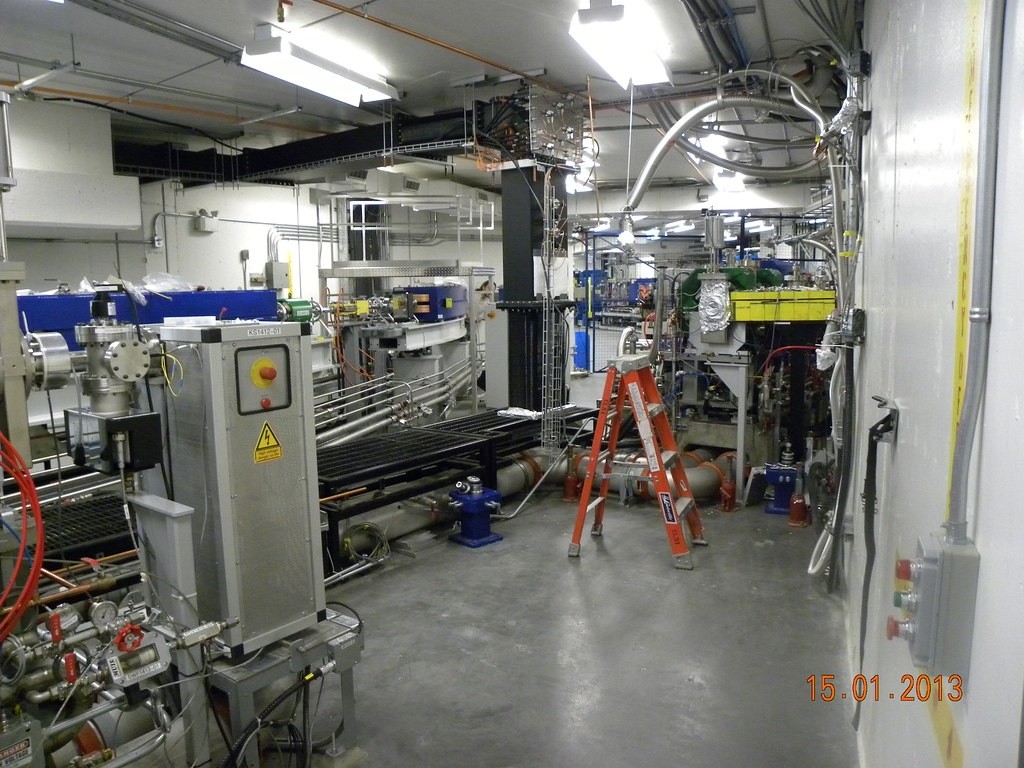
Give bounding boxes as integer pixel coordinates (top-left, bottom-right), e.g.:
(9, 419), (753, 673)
(568, 354), (708, 570)
